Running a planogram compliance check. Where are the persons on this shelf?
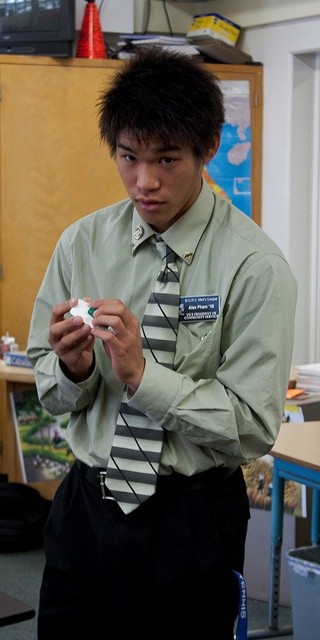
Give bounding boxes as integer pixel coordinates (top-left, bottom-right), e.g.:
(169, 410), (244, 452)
(25, 47), (298, 639)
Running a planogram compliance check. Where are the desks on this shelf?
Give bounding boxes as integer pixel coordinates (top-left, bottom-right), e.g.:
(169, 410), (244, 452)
(0, 361), (75, 501)
(235, 420), (320, 640)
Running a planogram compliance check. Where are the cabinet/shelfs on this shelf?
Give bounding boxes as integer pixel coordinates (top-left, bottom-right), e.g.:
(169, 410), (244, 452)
(0, 54), (264, 360)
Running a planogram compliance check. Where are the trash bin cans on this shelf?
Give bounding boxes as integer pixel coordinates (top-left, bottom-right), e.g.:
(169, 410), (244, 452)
(283, 546), (320, 640)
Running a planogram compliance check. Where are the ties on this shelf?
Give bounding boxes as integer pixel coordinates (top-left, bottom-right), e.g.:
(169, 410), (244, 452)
(105, 237), (181, 516)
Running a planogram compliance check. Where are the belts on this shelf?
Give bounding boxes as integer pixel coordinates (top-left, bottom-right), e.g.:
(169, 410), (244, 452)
(74, 459), (117, 502)
